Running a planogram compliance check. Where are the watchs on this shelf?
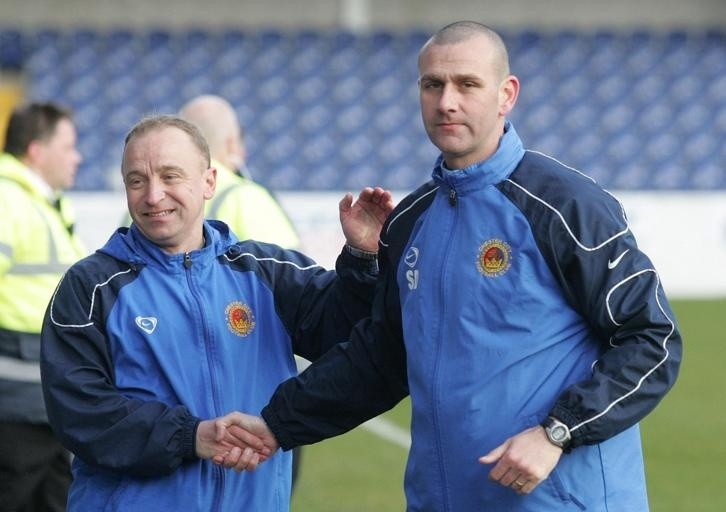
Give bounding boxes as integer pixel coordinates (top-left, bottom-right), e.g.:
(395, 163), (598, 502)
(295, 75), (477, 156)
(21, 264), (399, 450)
(537, 415), (576, 454)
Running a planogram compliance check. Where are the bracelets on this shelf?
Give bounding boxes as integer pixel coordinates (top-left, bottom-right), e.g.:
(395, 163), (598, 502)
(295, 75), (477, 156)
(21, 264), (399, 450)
(343, 243), (378, 261)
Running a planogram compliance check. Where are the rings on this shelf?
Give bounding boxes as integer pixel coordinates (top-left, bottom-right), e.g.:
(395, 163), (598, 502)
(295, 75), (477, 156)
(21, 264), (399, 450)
(514, 479), (524, 487)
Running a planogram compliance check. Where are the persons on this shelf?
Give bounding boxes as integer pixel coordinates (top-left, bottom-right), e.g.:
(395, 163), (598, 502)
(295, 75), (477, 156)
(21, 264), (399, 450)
(213, 20), (682, 512)
(38, 112), (396, 511)
(0, 101), (86, 512)
(119, 90), (305, 251)
